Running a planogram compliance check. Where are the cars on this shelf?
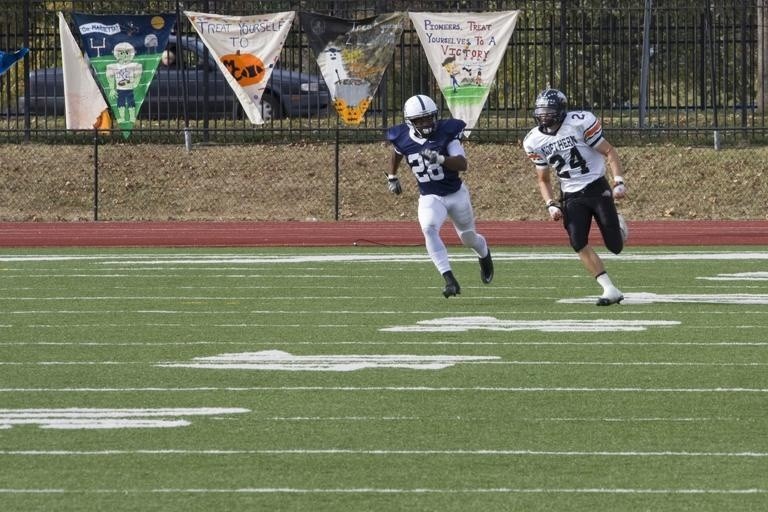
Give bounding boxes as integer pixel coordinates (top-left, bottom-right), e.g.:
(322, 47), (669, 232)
(19, 30), (332, 122)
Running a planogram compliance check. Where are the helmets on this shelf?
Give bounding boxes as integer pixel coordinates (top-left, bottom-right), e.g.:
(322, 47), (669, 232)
(404, 95), (437, 139)
(532, 88), (567, 127)
(113, 42), (135, 60)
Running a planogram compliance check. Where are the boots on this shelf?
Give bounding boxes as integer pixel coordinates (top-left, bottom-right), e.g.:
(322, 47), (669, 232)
(126, 107), (136, 122)
(116, 106), (126, 123)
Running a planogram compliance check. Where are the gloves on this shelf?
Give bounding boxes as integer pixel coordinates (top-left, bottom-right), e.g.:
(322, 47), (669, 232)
(613, 184), (624, 198)
(389, 178), (402, 194)
(548, 206), (561, 220)
(419, 150), (438, 163)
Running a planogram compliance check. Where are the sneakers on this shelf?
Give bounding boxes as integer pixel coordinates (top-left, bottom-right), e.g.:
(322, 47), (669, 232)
(478, 247), (493, 283)
(443, 283), (460, 298)
(618, 214), (628, 241)
(597, 287), (623, 307)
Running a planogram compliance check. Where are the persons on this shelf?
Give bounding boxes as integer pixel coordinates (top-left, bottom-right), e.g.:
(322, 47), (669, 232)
(520, 89), (629, 306)
(384, 93), (495, 298)
(162, 50), (176, 69)
(105, 41), (143, 124)
